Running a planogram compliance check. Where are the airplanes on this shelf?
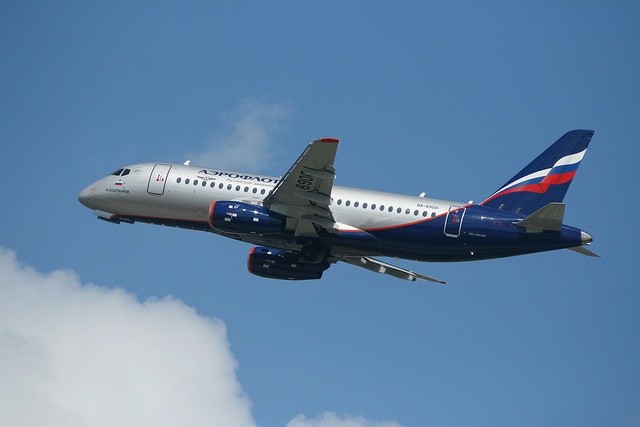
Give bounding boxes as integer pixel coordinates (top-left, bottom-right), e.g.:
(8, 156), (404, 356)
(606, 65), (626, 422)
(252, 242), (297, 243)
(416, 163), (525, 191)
(78, 127), (600, 285)
